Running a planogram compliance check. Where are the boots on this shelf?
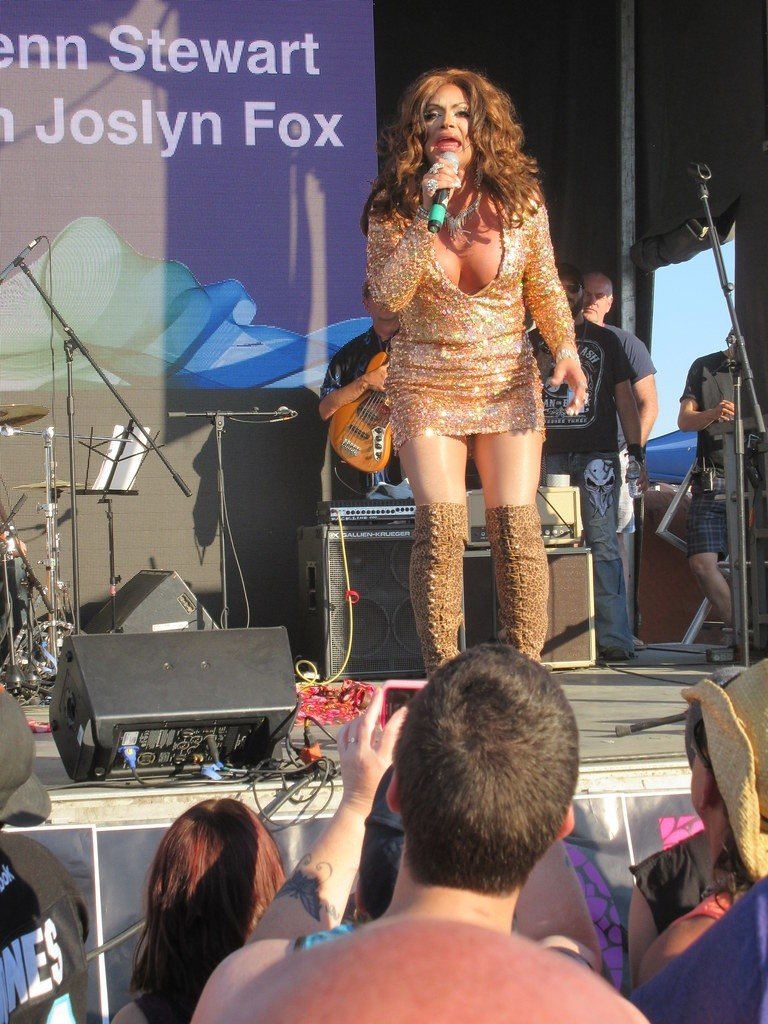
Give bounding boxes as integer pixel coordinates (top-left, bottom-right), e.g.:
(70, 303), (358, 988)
(483, 505), (548, 664)
(410, 504), (470, 681)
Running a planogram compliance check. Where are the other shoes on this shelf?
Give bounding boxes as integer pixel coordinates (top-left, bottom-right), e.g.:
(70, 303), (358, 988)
(720, 628), (743, 646)
(603, 646), (631, 661)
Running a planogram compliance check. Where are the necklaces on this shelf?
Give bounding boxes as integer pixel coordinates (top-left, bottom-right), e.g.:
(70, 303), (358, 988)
(445, 184), (482, 243)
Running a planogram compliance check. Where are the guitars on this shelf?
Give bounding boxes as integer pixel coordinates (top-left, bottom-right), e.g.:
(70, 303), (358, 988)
(329, 318), (400, 474)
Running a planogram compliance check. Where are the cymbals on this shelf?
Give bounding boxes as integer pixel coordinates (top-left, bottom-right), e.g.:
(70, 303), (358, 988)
(0, 404), (50, 427)
(13, 479), (85, 489)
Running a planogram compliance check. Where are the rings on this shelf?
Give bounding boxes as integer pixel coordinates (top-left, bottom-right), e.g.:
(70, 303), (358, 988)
(426, 179), (438, 192)
(429, 162), (444, 175)
(346, 737), (358, 744)
(573, 380), (588, 388)
(584, 392), (589, 404)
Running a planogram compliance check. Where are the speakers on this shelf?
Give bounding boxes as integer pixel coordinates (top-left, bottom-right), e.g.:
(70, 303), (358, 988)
(47, 569), (299, 783)
(459, 548), (597, 668)
(466, 486), (581, 547)
(298, 524), (428, 680)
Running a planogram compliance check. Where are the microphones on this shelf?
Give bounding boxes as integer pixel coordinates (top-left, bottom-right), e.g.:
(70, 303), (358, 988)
(277, 406), (298, 416)
(428, 151), (460, 233)
(0, 236), (43, 284)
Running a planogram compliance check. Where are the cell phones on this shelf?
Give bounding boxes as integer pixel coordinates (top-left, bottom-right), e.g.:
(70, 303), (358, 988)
(379, 680), (428, 732)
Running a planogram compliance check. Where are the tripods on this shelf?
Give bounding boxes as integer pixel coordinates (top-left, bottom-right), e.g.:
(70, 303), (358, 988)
(0, 493), (53, 706)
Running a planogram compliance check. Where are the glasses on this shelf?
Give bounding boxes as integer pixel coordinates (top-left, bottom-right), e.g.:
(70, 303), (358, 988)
(693, 717), (714, 777)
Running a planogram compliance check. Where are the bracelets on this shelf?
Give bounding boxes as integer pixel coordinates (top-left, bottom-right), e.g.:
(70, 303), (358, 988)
(555, 348), (580, 363)
(417, 205), (429, 218)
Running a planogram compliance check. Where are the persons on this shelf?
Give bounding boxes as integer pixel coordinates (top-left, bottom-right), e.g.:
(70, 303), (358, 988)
(0, 689), (90, 1024)
(319, 67), (768, 679)
(110, 642), (768, 1023)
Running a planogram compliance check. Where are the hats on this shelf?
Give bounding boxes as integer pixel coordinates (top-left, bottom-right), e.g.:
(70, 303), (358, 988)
(680, 659), (768, 883)
(1, 685), (52, 827)
